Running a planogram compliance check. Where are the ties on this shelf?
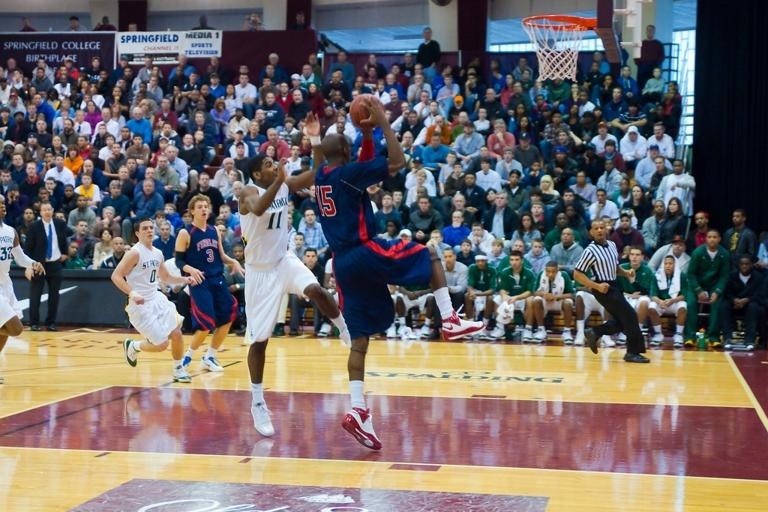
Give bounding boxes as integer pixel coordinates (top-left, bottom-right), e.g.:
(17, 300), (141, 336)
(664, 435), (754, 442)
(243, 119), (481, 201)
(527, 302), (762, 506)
(46, 224), (52, 260)
(77, 126), (80, 133)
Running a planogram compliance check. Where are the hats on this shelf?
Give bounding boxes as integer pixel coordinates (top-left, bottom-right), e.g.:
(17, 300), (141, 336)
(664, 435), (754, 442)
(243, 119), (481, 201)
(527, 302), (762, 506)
(412, 68), (658, 176)
(290, 73), (301, 80)
(234, 127), (243, 133)
(158, 136), (168, 142)
(669, 235), (687, 243)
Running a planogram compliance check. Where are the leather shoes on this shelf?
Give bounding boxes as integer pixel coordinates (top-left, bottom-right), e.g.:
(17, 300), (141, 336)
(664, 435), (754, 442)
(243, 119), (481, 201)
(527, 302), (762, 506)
(583, 329), (597, 354)
(624, 353), (648, 363)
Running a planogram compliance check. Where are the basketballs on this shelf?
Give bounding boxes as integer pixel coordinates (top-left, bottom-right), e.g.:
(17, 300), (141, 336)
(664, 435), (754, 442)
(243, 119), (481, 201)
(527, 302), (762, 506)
(351, 94), (384, 131)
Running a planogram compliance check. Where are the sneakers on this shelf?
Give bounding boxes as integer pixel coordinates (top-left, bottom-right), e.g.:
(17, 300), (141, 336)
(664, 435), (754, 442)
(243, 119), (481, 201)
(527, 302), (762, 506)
(466, 326), (614, 347)
(123, 339), (140, 366)
(438, 311), (483, 341)
(182, 354), (224, 371)
(386, 324), (429, 339)
(172, 365), (191, 383)
(341, 407), (380, 450)
(615, 339), (625, 345)
(339, 328), (351, 348)
(651, 332), (754, 351)
(250, 401), (274, 436)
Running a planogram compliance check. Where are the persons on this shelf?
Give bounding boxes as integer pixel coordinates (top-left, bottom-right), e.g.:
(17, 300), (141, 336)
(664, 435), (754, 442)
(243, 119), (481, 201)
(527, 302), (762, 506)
(311, 93), (486, 451)
(1, 12), (766, 352)
(109, 217), (195, 384)
(236, 110), (352, 438)
(0, 198), (46, 384)
(173, 194), (246, 373)
(571, 217), (651, 364)
